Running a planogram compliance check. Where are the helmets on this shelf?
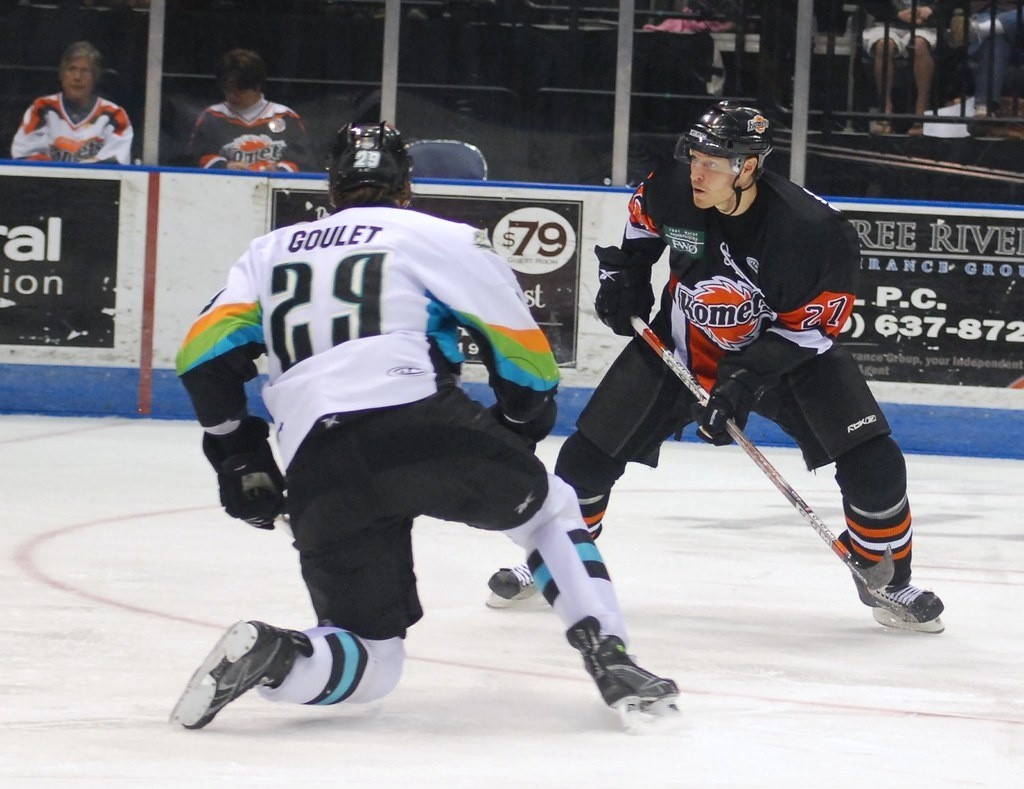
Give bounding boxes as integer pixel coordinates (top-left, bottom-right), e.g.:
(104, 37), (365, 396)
(327, 119), (415, 196)
(683, 99), (776, 158)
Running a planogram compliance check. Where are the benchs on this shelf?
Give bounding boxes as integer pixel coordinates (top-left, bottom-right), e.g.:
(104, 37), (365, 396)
(500, 0), (1024, 95)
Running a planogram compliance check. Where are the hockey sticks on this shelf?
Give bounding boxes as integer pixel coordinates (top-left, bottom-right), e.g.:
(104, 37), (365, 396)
(633, 316), (899, 593)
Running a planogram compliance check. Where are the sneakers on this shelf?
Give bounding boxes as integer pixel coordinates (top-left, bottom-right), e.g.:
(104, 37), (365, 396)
(566, 615), (681, 726)
(850, 565), (945, 633)
(482, 561), (539, 607)
(170, 619), (314, 729)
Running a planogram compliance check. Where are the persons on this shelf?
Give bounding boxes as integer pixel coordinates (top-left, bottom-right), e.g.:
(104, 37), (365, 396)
(11, 39), (135, 166)
(188, 49), (320, 174)
(861, 0), (1023, 137)
(173, 122), (681, 737)
(485, 100), (945, 635)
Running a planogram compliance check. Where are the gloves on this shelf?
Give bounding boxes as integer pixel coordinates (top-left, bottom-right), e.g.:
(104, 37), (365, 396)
(484, 399), (560, 454)
(201, 415), (291, 531)
(591, 244), (656, 337)
(696, 362), (775, 446)
